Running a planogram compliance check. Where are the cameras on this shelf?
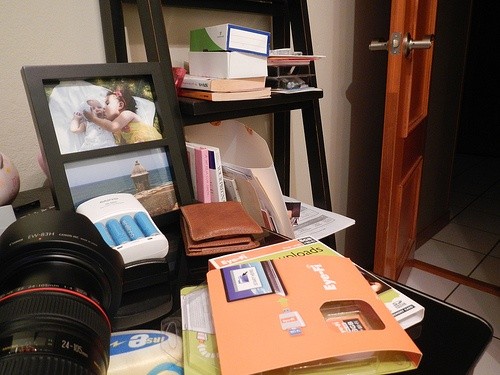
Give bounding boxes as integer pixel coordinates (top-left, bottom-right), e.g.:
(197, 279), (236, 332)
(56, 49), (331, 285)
(0, 210), (126, 375)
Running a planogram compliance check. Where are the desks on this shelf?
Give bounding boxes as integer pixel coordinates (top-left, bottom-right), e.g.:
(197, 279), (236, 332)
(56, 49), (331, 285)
(12, 183), (494, 375)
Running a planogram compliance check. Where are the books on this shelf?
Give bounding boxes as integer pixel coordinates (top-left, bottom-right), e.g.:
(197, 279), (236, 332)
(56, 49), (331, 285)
(283, 195), (355, 240)
(208, 235), (425, 330)
(173, 22), (327, 102)
(184, 143), (242, 205)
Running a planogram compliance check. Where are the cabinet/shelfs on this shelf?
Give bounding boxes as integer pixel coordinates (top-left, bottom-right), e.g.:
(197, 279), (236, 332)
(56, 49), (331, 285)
(94, 0), (338, 250)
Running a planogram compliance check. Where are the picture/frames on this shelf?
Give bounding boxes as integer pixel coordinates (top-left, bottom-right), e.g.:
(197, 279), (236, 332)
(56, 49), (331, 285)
(19, 59), (189, 226)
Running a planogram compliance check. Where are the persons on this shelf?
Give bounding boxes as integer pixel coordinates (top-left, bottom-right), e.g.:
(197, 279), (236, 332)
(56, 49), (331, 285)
(67, 86), (162, 146)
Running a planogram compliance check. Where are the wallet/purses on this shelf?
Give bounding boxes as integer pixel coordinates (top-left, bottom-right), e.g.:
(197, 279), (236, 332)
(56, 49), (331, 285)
(179, 201), (264, 256)
